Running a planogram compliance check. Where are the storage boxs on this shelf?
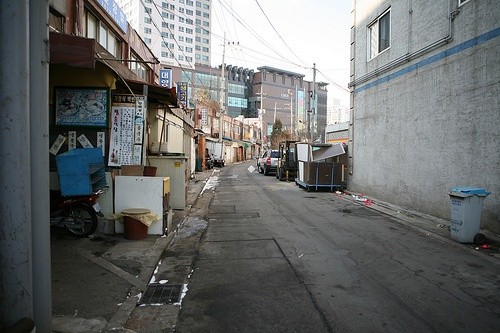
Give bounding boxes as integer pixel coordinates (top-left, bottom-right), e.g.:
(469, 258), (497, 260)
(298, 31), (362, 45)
(121, 165), (144, 176)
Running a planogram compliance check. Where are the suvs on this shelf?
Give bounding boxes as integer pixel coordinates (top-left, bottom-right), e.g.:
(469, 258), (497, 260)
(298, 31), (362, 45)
(256, 149), (286, 176)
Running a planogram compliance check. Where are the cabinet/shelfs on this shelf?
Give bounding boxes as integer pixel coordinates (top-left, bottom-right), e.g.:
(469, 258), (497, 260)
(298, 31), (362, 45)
(56, 148), (107, 195)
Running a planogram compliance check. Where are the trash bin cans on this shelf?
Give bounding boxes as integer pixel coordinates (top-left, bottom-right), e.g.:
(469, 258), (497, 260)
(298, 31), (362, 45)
(448, 186), (491, 243)
(196, 159), (203, 172)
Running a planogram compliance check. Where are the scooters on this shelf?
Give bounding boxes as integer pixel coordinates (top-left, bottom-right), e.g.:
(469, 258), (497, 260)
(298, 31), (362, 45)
(50, 185), (110, 238)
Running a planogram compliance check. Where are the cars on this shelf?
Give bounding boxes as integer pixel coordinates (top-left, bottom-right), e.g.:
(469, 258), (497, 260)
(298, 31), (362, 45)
(276, 147), (298, 182)
(194, 147), (226, 170)
